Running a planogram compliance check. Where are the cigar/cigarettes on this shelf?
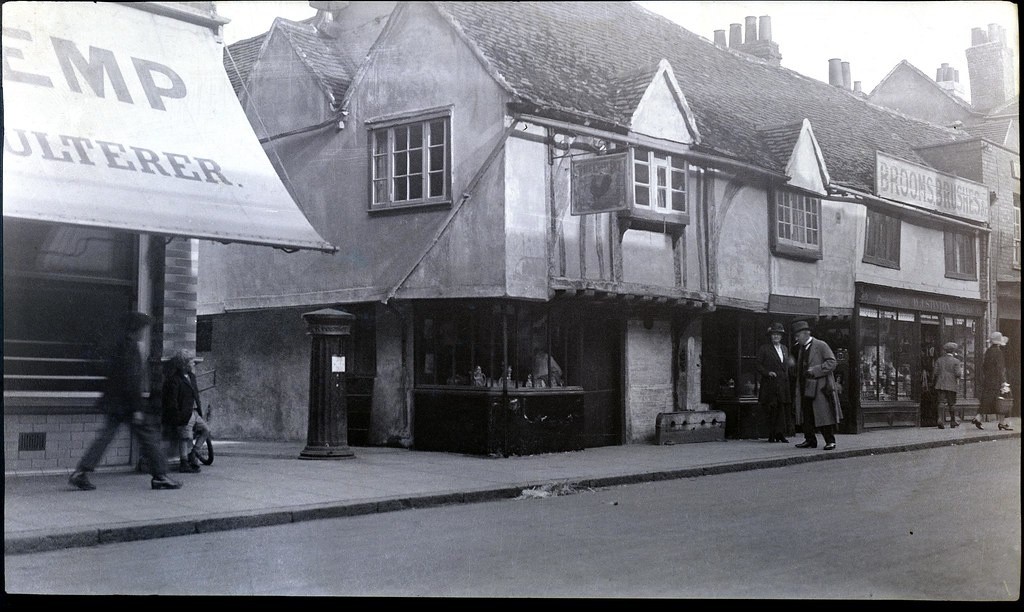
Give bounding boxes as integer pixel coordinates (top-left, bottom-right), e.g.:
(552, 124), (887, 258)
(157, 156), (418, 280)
(795, 341), (799, 346)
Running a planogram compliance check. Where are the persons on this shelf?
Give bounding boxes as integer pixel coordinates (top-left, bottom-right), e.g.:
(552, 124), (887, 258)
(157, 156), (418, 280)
(972, 331), (1014, 430)
(530, 342), (563, 386)
(756, 323), (794, 442)
(68, 309), (184, 490)
(933, 341), (961, 429)
(161, 347), (209, 473)
(785, 321), (843, 451)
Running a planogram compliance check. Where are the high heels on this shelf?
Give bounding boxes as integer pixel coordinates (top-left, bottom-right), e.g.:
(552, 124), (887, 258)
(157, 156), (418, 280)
(998, 423), (1013, 430)
(767, 432), (790, 444)
(972, 418), (984, 429)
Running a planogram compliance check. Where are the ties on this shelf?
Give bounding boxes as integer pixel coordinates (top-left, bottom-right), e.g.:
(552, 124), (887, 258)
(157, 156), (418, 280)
(802, 345), (806, 372)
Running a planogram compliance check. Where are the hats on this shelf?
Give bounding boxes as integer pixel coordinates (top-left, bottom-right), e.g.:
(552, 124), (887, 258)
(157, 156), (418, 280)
(766, 323), (784, 333)
(124, 311), (151, 324)
(942, 342), (957, 349)
(986, 332), (1009, 346)
(790, 321), (809, 334)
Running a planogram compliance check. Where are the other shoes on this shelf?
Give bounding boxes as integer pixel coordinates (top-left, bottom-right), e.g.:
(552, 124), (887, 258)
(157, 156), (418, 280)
(937, 420), (945, 429)
(179, 454), (200, 473)
(951, 422), (959, 427)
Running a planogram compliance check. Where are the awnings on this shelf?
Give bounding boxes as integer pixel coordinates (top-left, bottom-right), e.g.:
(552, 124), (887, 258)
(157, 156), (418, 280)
(1, 0), (341, 254)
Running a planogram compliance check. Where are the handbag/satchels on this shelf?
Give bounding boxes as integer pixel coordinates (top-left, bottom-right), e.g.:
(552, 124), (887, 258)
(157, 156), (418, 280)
(805, 375), (817, 398)
(996, 388), (1014, 413)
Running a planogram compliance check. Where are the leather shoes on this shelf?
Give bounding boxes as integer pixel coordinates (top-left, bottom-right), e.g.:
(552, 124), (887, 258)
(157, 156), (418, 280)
(71, 472), (96, 490)
(796, 440), (817, 448)
(824, 442), (836, 449)
(153, 477), (182, 490)
